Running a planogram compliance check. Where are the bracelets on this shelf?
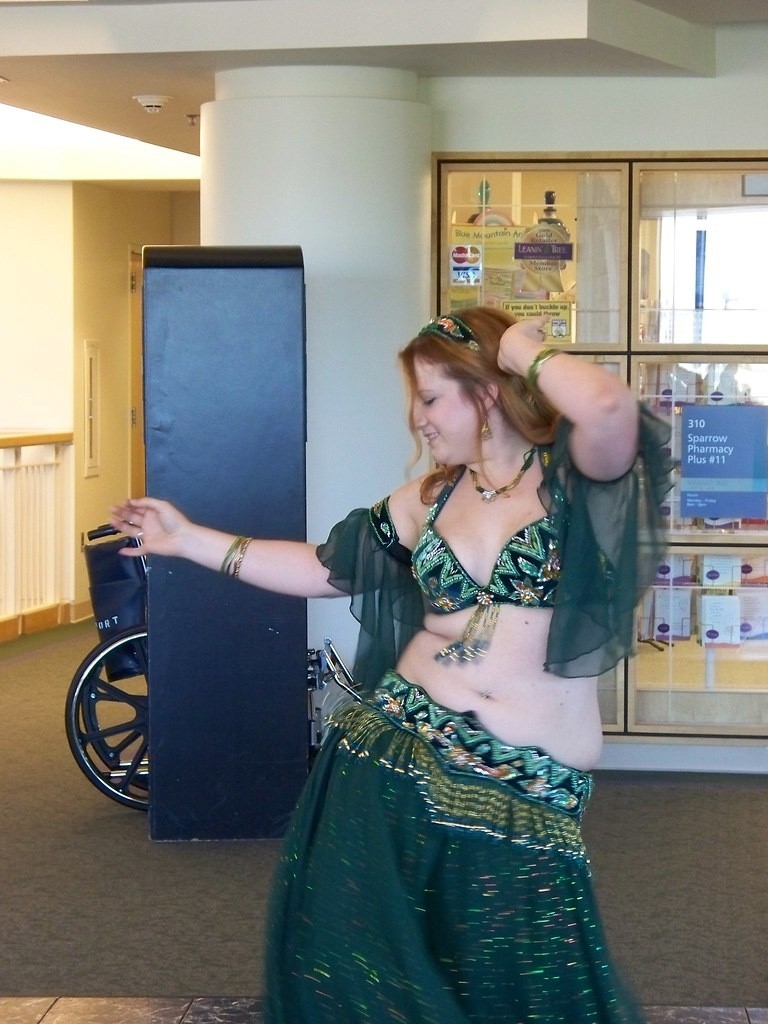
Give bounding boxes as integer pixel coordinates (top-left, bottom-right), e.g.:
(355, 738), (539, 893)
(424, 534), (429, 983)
(530, 347), (566, 393)
(219, 537), (253, 580)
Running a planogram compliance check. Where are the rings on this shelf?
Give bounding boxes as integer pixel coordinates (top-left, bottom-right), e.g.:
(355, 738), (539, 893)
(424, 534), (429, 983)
(136, 530), (143, 538)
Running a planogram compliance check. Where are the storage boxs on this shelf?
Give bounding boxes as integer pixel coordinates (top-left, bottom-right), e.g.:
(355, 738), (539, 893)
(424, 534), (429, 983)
(633, 361), (768, 646)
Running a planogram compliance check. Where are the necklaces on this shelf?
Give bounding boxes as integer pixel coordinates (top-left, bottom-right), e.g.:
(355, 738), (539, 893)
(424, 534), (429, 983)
(470, 442), (534, 505)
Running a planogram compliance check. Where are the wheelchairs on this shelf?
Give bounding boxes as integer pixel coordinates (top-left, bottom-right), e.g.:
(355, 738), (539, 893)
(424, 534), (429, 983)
(65, 521), (364, 815)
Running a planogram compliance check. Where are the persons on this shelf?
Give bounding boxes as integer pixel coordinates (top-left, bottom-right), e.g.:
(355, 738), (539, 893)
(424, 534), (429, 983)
(109, 307), (683, 1024)
